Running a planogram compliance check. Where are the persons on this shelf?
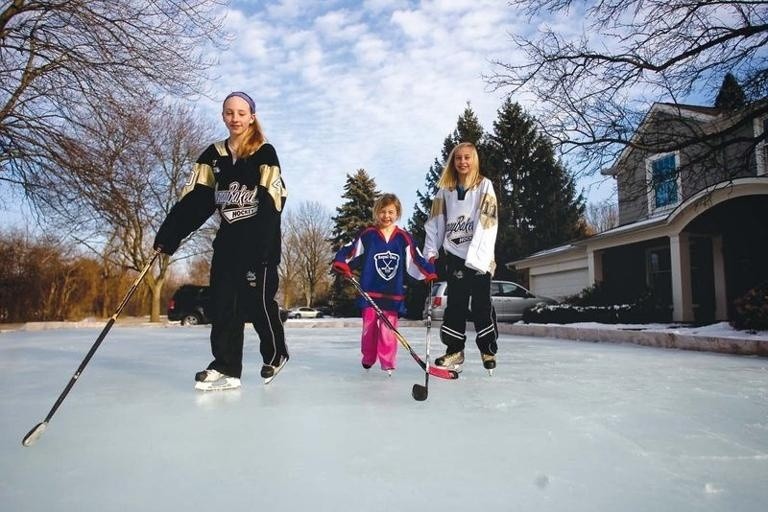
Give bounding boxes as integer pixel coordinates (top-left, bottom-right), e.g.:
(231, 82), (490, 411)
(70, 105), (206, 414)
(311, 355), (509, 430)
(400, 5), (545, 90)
(332, 193), (438, 369)
(422, 141), (499, 369)
(153, 92), (289, 383)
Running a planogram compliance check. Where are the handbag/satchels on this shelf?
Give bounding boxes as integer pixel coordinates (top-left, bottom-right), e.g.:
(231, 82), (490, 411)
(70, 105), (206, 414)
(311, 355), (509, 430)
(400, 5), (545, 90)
(436, 247), (477, 280)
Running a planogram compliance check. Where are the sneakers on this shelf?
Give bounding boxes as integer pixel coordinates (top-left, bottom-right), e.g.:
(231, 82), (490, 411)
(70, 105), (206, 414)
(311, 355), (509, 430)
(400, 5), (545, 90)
(481, 352), (495, 369)
(195, 369), (240, 392)
(262, 355), (291, 385)
(435, 351), (464, 365)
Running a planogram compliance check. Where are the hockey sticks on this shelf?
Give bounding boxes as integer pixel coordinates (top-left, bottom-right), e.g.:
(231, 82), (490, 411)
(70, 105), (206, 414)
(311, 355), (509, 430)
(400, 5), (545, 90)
(346, 274), (458, 380)
(23, 245), (163, 447)
(412, 279), (435, 401)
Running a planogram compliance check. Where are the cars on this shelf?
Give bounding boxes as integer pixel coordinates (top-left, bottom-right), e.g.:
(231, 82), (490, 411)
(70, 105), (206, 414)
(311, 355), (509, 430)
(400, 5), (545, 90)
(421, 280), (560, 322)
(287, 307), (323, 319)
(166, 284), (212, 326)
